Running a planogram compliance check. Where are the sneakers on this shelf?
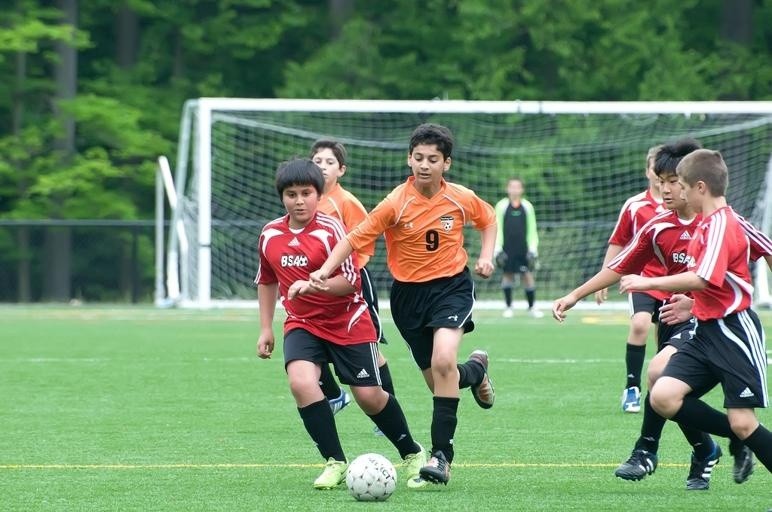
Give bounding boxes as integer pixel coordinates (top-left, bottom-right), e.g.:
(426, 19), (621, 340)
(686, 439), (722, 490)
(329, 388), (351, 415)
(468, 350), (495, 409)
(623, 386), (641, 413)
(420, 450), (450, 486)
(401, 441), (425, 488)
(615, 449), (657, 480)
(314, 457), (348, 490)
(734, 445), (756, 483)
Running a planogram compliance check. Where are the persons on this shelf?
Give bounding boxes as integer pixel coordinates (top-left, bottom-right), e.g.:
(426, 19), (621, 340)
(619, 148), (772, 473)
(595, 143), (669, 412)
(306, 139), (396, 436)
(493, 178), (545, 318)
(252, 160), (425, 490)
(307, 122), (499, 486)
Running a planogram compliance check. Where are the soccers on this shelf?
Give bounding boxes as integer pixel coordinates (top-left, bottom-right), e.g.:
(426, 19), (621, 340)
(344, 452), (398, 501)
